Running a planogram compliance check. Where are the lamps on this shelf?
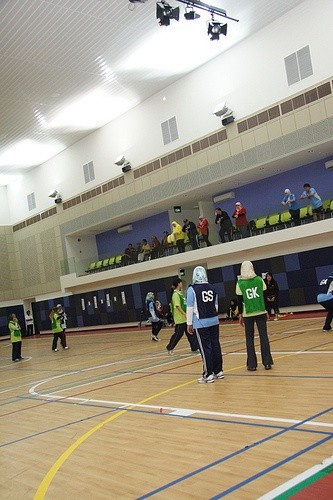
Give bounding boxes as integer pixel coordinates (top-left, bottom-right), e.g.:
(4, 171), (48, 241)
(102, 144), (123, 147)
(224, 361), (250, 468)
(212, 101), (235, 126)
(113, 155), (132, 173)
(127, 0), (228, 41)
(48, 190), (62, 203)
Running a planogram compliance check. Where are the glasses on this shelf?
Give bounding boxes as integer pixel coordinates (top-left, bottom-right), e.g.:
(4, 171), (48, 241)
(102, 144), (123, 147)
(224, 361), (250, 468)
(235, 205), (241, 207)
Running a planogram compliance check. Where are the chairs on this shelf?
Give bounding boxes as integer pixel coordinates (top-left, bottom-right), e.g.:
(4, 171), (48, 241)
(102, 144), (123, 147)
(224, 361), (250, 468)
(224, 198), (333, 242)
(84, 230), (208, 275)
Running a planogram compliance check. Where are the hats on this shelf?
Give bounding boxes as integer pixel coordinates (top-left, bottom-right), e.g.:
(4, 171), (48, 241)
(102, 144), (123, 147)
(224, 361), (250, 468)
(56, 304), (62, 308)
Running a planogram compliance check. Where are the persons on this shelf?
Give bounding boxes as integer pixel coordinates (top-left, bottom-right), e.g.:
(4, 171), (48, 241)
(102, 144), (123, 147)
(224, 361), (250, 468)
(280, 189), (302, 227)
(236, 260), (273, 371)
(8, 313), (25, 362)
(299, 183), (324, 221)
(263, 273), (279, 321)
(231, 201), (251, 240)
(166, 278), (201, 356)
(49, 307), (69, 352)
(214, 207), (233, 243)
(25, 310), (34, 337)
(186, 266), (224, 383)
(317, 272), (333, 332)
(121, 231), (170, 266)
(182, 219), (199, 250)
(227, 298), (239, 321)
(196, 216), (212, 247)
(172, 221), (186, 254)
(56, 304), (67, 348)
(145, 292), (175, 342)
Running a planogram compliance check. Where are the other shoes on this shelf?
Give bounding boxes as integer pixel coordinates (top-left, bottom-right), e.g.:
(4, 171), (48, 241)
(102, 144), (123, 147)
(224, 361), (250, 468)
(65, 347), (69, 350)
(152, 336), (159, 342)
(274, 318), (279, 321)
(15, 359), (19, 362)
(192, 349), (201, 353)
(323, 328), (333, 332)
(21, 357), (24, 359)
(165, 345), (174, 355)
(54, 349), (58, 352)
(247, 366), (256, 371)
(265, 365), (271, 369)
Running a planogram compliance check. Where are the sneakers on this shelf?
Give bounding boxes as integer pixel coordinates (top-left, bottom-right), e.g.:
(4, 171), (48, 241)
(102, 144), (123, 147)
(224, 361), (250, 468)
(214, 371), (225, 380)
(197, 374), (214, 384)
